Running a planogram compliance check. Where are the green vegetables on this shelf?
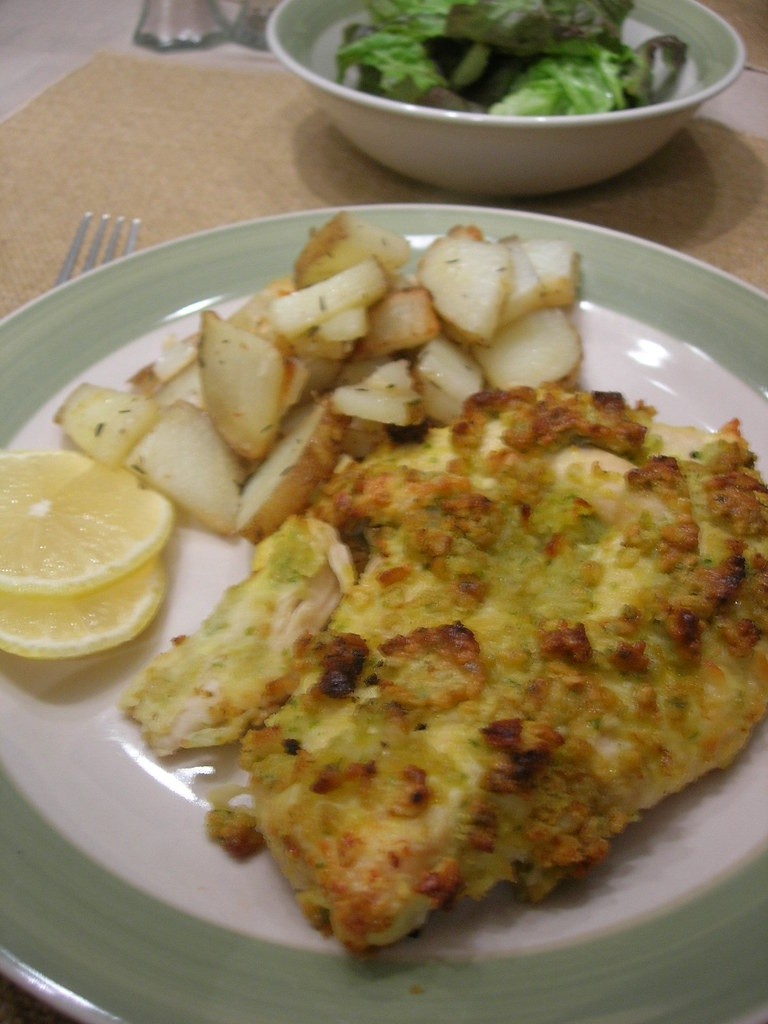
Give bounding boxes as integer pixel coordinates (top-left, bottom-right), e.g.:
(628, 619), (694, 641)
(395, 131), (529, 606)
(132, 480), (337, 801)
(334, 1), (634, 117)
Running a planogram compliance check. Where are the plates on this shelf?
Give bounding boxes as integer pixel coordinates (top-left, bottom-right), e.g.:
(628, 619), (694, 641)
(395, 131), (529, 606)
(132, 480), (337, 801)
(0, 206), (768, 1022)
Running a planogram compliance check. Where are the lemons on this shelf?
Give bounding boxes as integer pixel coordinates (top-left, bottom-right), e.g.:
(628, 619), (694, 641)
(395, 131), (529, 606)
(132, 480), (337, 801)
(0, 449), (175, 663)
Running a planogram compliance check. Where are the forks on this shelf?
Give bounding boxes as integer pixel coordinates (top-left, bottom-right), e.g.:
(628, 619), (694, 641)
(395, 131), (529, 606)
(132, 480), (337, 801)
(49, 213), (142, 296)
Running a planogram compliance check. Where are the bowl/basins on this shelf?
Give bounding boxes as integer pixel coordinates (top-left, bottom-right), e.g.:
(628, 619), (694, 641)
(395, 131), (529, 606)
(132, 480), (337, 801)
(263, 1), (746, 197)
